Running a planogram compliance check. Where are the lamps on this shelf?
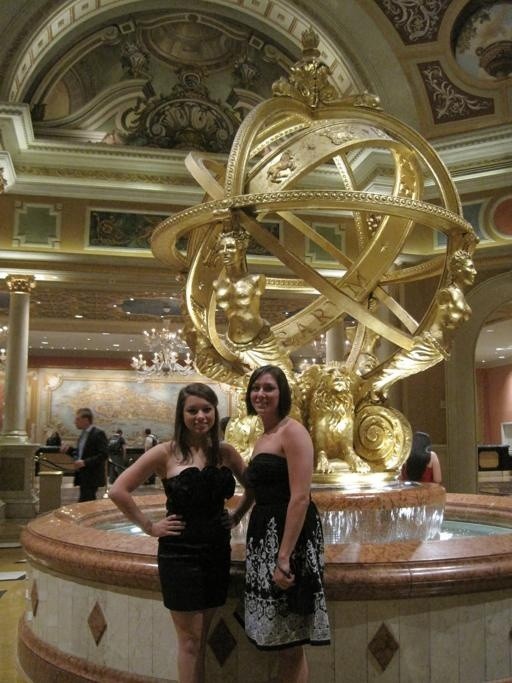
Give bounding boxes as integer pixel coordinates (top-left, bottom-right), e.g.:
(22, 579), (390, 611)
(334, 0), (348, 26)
(296, 333), (350, 378)
(128, 318), (197, 384)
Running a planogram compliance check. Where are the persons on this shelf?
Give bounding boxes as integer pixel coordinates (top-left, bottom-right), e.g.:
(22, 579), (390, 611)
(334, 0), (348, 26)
(141, 426), (160, 485)
(204, 229), (293, 368)
(218, 417), (231, 440)
(108, 380), (256, 682)
(44, 429), (62, 446)
(105, 428), (127, 484)
(58, 406), (110, 501)
(360, 248), (481, 390)
(234, 365), (331, 682)
(399, 430), (445, 487)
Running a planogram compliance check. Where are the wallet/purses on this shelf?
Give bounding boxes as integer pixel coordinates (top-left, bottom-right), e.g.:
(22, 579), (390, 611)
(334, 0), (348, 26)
(274, 585), (300, 609)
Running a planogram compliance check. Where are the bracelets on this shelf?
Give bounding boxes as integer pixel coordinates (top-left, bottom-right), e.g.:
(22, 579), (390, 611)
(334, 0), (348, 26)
(142, 518), (153, 535)
